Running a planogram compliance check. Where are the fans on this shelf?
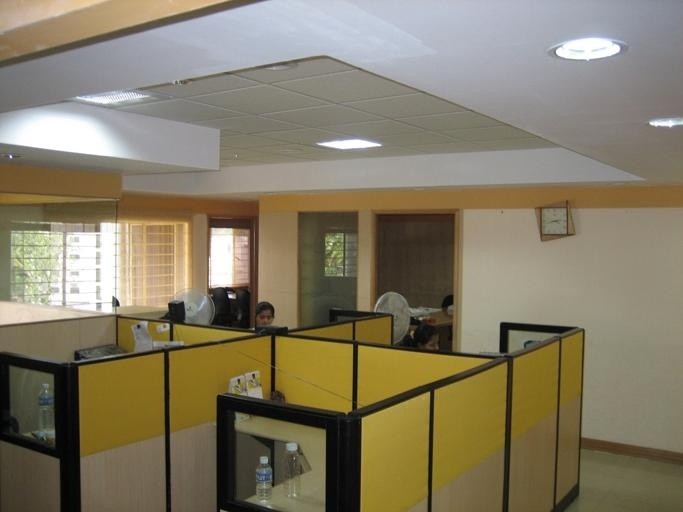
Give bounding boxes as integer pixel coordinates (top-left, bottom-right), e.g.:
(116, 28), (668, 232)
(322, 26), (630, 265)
(176, 288), (217, 325)
(375, 290), (413, 346)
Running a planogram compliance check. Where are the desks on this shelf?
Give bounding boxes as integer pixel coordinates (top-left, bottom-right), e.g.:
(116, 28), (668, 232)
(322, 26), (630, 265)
(216, 410), (326, 512)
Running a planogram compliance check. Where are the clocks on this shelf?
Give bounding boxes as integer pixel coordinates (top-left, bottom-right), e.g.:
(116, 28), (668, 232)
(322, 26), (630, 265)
(534, 199), (575, 241)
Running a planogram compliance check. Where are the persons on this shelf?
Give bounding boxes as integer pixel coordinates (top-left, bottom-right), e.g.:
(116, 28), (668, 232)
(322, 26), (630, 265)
(255, 300), (274, 329)
(412, 322), (441, 352)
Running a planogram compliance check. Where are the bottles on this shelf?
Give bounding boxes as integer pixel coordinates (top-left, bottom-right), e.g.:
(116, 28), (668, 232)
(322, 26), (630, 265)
(37, 383), (55, 439)
(255, 455), (273, 509)
(282, 440), (302, 499)
